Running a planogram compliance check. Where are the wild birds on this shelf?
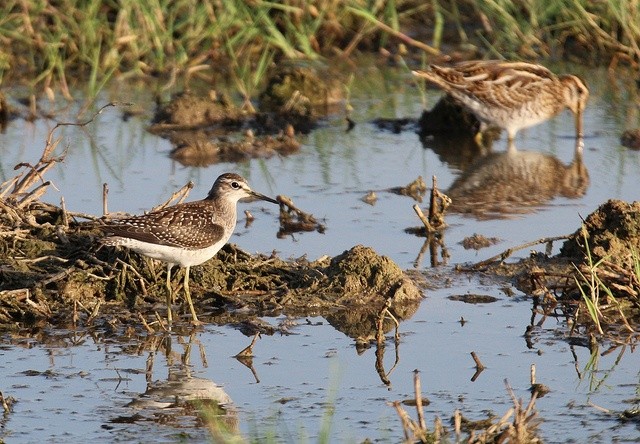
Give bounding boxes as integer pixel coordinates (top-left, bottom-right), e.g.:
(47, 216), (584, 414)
(435, 150), (590, 222)
(91, 172), (280, 333)
(121, 332), (242, 440)
(408, 57), (590, 163)
